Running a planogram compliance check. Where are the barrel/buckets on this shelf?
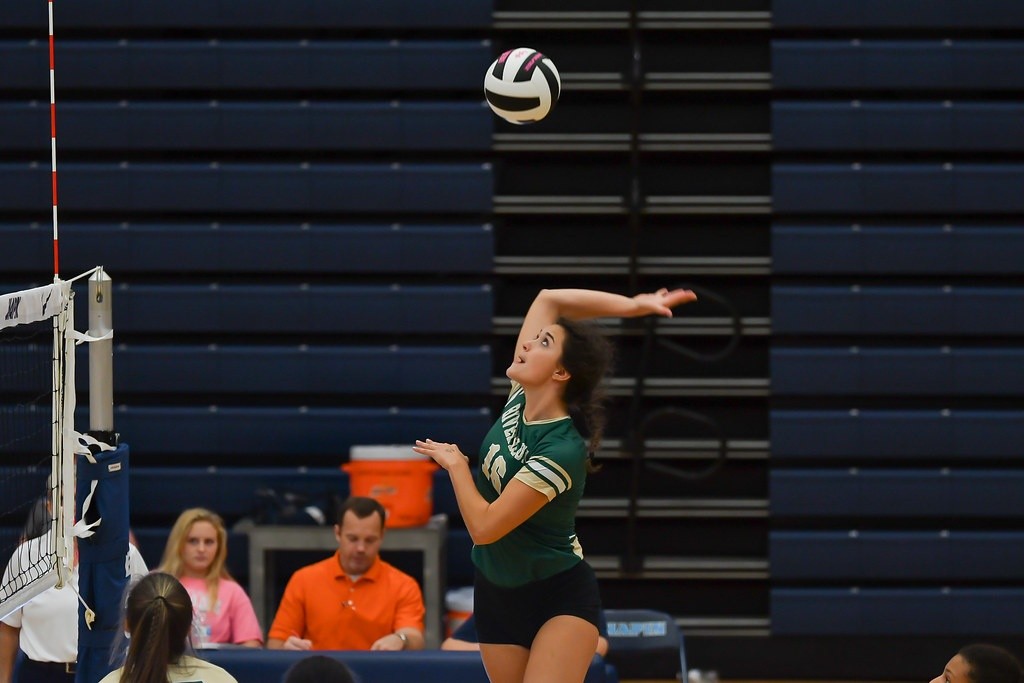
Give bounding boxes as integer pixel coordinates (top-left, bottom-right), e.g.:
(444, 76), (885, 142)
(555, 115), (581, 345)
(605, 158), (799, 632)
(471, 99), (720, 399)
(443, 584), (475, 640)
(342, 455), (439, 528)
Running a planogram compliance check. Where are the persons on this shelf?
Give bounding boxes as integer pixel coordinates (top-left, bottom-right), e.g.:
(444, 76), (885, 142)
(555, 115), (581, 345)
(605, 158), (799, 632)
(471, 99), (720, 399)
(1, 469), (149, 683)
(20, 459), (139, 549)
(97, 573), (239, 682)
(412, 288), (699, 680)
(281, 655), (357, 683)
(148, 506), (264, 648)
(265, 496), (425, 652)
(929, 640), (1024, 683)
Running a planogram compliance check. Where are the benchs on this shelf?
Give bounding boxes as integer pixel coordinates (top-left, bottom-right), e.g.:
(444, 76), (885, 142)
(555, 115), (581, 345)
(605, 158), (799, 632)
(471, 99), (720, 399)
(3, 3), (1022, 637)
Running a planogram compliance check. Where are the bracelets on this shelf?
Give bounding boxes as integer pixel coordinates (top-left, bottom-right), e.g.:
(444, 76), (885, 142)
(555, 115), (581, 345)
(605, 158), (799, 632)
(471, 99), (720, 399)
(395, 632), (406, 646)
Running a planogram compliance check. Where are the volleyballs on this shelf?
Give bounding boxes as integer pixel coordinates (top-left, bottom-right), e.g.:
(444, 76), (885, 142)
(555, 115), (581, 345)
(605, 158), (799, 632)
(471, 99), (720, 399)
(481, 46), (565, 128)
